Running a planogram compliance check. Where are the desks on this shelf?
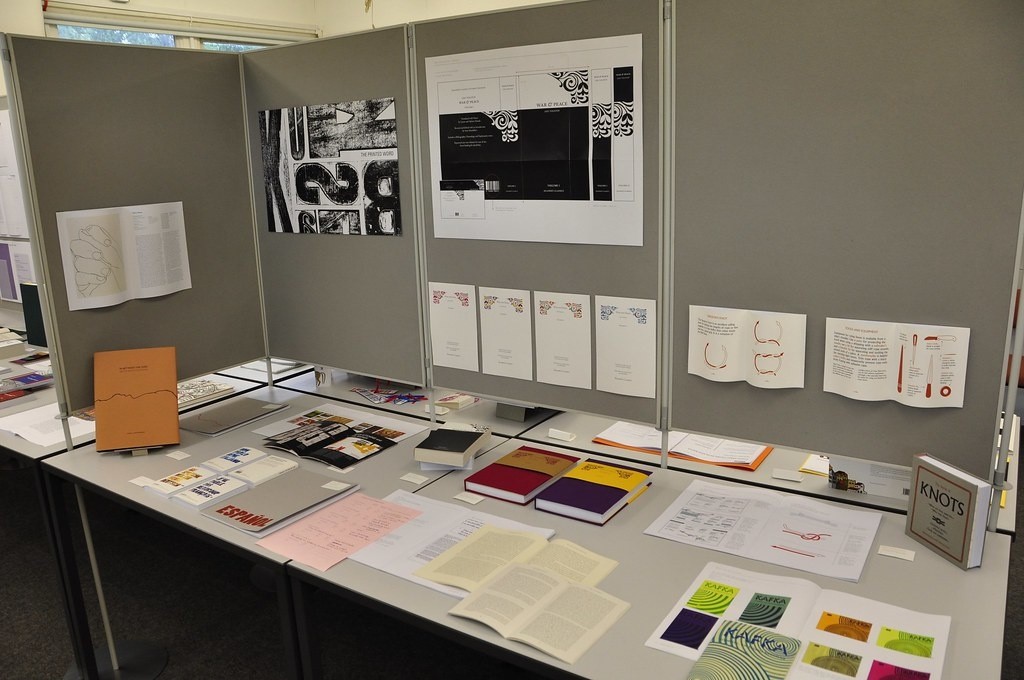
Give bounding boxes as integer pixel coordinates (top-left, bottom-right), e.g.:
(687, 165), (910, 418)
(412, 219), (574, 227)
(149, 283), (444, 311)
(41, 384), (509, 680)
(0, 327), (315, 680)
(518, 411), (1019, 542)
(274, 368), (561, 437)
(285, 436), (1011, 680)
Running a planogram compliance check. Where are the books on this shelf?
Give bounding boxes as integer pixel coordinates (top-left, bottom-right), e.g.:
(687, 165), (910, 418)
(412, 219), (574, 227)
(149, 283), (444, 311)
(434, 392), (480, 410)
(93, 347), (291, 454)
(905, 453), (993, 571)
(0, 281), (55, 411)
(644, 560), (948, 680)
(147, 446), (360, 539)
(414, 419), (491, 470)
(535, 457), (653, 527)
(592, 419), (775, 472)
(464, 444), (581, 506)
(414, 524), (630, 665)
